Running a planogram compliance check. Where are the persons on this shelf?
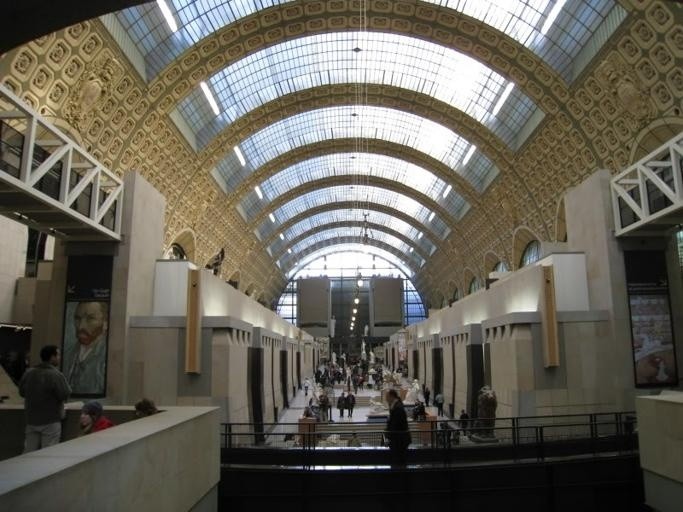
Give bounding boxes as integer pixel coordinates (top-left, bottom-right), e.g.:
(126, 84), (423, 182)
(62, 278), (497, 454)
(435, 391), (444, 417)
(459, 409), (469, 436)
(412, 399), (427, 421)
(19, 344), (72, 454)
(346, 391), (355, 417)
(284, 434), (295, 442)
(304, 355), (385, 390)
(80, 401), (115, 434)
(62, 302), (108, 398)
(424, 387), (430, 406)
(337, 392), (346, 418)
(385, 388), (412, 469)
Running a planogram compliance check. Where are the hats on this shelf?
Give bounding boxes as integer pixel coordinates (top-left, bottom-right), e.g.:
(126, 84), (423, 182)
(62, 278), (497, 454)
(79, 400), (103, 434)
